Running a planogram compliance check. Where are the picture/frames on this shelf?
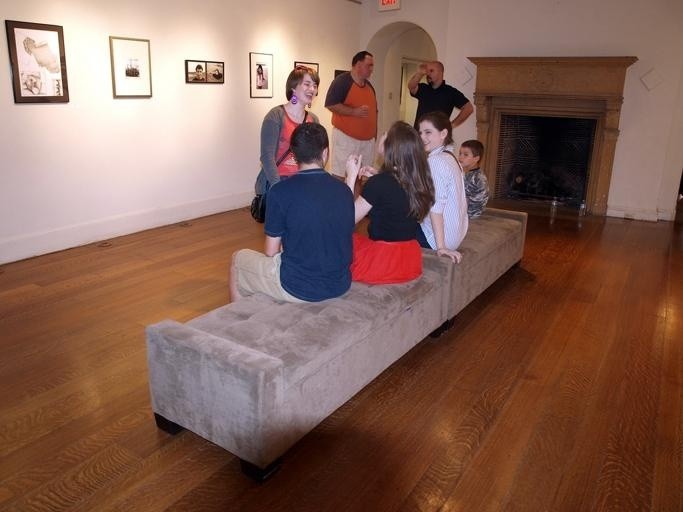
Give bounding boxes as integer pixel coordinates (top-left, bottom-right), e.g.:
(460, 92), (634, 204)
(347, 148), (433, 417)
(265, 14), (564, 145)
(294, 61), (319, 96)
(206, 60), (224, 84)
(249, 52), (273, 98)
(185, 59), (207, 83)
(334, 70), (351, 79)
(109, 35), (152, 98)
(4, 19), (69, 104)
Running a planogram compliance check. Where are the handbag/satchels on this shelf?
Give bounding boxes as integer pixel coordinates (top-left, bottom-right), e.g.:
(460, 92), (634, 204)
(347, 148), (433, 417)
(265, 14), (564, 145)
(250, 110), (309, 224)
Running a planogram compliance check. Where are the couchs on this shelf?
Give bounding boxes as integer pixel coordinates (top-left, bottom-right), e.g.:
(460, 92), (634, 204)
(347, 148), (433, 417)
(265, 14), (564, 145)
(339, 206), (529, 333)
(143, 260), (448, 485)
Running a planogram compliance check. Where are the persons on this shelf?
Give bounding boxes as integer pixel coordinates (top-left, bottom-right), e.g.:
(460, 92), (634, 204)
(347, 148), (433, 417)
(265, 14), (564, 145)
(213, 69), (223, 80)
(323, 51), (378, 199)
(193, 65), (205, 82)
(256, 65), (265, 90)
(416, 112), (469, 265)
(344, 121), (433, 284)
(228, 123), (357, 303)
(407, 61), (474, 131)
(254, 66), (320, 195)
(458, 140), (490, 221)
